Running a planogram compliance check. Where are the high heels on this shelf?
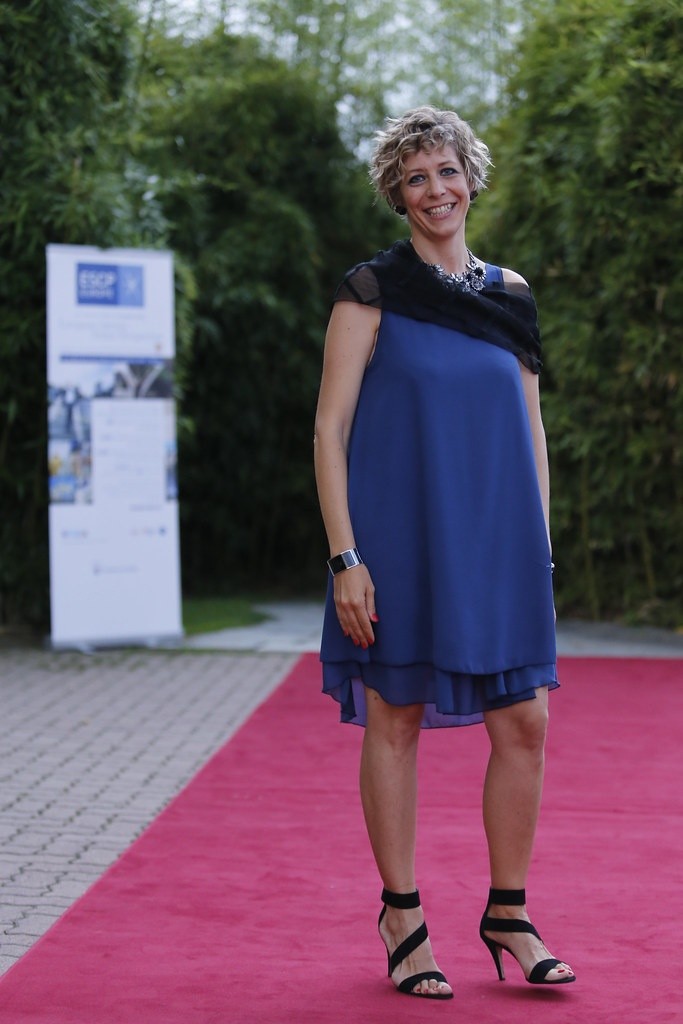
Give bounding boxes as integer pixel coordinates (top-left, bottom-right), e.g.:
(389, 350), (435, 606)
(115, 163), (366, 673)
(378, 886), (455, 1000)
(479, 886), (576, 984)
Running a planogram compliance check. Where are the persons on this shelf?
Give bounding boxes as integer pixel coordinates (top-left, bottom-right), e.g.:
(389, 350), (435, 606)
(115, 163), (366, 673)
(313, 103), (577, 999)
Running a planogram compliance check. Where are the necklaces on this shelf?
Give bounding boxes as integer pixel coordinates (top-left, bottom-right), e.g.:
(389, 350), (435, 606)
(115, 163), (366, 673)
(421, 242), (486, 296)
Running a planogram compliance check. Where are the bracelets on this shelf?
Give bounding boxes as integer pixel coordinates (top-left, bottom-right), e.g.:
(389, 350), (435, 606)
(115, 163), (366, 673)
(327, 548), (364, 576)
